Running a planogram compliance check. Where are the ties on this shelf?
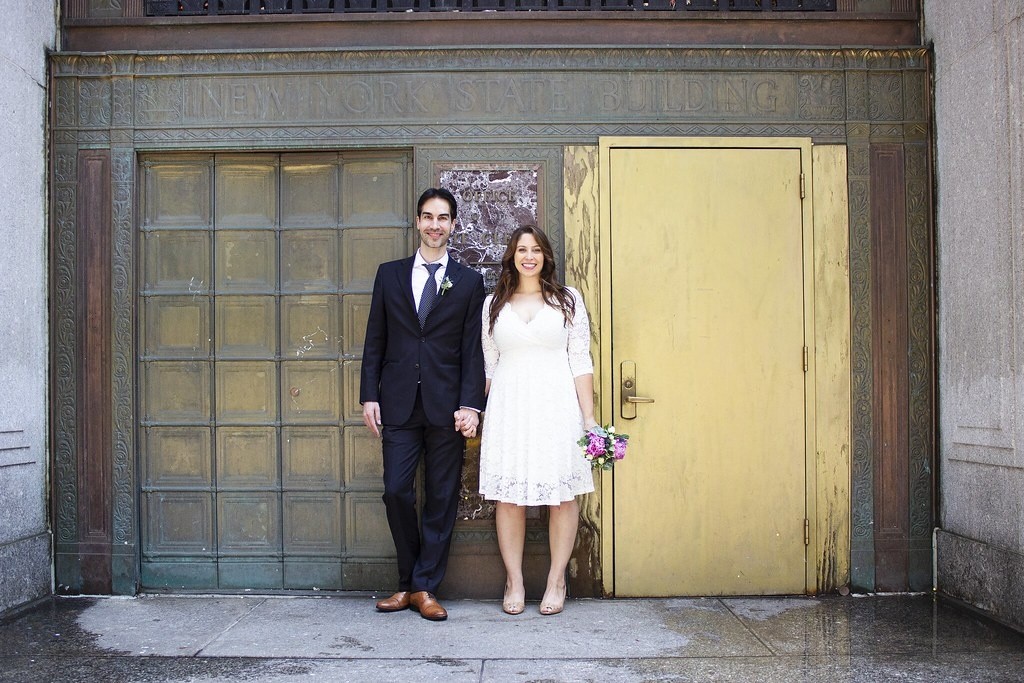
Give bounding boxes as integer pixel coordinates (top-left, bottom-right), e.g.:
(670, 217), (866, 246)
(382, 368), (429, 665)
(418, 262), (442, 330)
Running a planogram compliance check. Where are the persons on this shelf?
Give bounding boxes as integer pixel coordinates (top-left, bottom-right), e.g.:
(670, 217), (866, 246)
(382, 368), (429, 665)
(454, 226), (609, 616)
(360, 188), (486, 620)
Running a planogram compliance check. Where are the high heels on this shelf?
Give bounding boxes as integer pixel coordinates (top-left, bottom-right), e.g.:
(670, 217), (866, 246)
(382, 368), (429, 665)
(541, 581), (567, 615)
(503, 579), (526, 614)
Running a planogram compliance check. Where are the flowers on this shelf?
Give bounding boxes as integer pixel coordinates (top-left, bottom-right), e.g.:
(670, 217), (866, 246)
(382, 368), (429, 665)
(576, 423), (629, 471)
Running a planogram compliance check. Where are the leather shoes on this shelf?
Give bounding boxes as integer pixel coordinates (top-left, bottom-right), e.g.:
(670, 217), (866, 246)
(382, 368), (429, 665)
(409, 593), (449, 620)
(376, 590), (412, 611)
